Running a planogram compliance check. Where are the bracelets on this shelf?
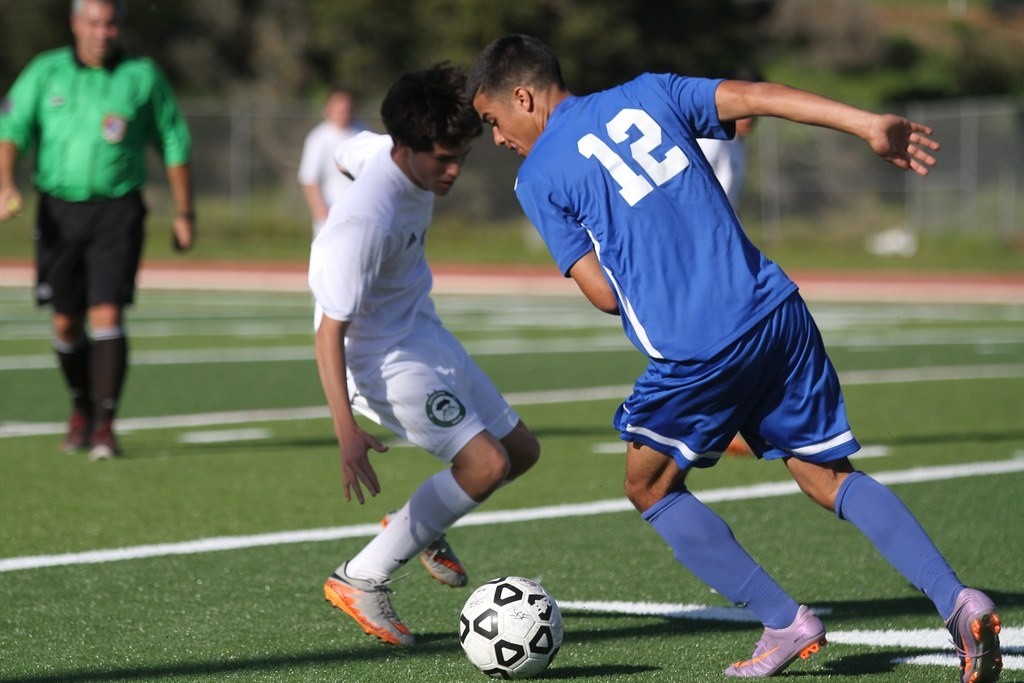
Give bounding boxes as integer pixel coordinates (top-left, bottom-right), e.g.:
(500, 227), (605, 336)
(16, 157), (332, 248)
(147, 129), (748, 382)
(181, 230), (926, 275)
(176, 211), (193, 221)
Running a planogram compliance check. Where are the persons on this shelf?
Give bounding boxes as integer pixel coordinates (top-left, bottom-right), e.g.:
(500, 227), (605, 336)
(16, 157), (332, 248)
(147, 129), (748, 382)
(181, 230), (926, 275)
(468, 35), (1003, 683)
(696, 67), (769, 212)
(296, 89), (357, 240)
(0, 0), (197, 461)
(306, 60), (542, 647)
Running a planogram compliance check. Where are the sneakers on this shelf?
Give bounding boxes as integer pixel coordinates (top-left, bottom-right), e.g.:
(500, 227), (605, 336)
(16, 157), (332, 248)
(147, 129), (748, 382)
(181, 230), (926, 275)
(323, 560), (416, 648)
(725, 604), (828, 678)
(382, 506), (468, 588)
(944, 588), (1003, 683)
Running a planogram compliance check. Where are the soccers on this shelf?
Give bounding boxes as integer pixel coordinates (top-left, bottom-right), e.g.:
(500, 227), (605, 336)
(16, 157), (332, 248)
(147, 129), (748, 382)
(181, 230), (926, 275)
(458, 575), (564, 681)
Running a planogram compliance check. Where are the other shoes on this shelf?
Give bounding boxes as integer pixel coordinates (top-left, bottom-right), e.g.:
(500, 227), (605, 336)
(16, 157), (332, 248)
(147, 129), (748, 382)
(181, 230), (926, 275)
(58, 415), (90, 455)
(88, 418), (114, 460)
